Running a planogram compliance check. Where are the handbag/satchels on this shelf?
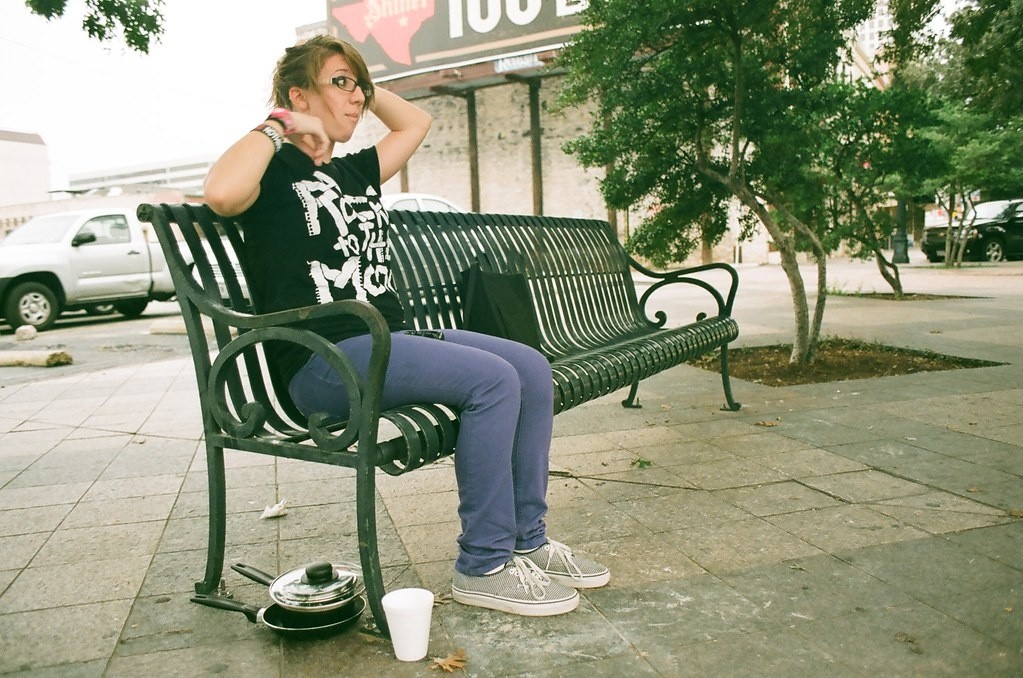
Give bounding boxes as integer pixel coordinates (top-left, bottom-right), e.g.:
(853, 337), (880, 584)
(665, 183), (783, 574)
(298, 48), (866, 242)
(457, 262), (543, 355)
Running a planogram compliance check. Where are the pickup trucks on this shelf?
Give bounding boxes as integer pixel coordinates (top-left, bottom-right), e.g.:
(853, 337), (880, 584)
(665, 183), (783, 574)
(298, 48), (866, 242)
(0, 194), (203, 332)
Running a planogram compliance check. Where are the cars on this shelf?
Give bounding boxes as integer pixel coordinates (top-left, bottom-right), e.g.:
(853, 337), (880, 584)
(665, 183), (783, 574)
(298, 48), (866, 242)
(919, 198), (1023, 262)
(190, 191), (508, 307)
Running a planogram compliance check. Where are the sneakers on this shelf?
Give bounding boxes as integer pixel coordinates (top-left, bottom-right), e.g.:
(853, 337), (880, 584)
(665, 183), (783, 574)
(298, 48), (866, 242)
(452, 556), (579, 616)
(513, 538), (610, 588)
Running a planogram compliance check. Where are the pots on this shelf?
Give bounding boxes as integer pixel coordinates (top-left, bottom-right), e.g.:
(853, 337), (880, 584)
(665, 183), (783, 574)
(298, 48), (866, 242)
(190, 593), (367, 637)
(230, 561), (366, 613)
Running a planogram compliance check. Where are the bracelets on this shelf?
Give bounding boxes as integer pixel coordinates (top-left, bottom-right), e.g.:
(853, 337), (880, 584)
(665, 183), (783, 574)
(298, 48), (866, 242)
(250, 124), (282, 152)
(265, 117), (286, 130)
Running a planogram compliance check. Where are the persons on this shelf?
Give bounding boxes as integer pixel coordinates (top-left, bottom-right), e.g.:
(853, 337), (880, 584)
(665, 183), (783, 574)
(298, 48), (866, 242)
(203, 34), (610, 617)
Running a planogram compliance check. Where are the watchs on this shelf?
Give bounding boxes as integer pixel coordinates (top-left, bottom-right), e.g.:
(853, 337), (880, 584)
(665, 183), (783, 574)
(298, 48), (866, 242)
(270, 108), (297, 137)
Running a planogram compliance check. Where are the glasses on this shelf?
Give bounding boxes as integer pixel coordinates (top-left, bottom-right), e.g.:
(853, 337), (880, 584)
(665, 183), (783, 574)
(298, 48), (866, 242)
(317, 75), (373, 96)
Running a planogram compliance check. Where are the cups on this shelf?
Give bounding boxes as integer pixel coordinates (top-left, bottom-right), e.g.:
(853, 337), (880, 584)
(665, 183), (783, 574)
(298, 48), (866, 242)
(380, 588), (434, 662)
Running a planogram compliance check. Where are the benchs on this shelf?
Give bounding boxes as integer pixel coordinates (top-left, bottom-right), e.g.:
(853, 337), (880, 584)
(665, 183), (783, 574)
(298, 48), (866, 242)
(138, 201), (743, 641)
